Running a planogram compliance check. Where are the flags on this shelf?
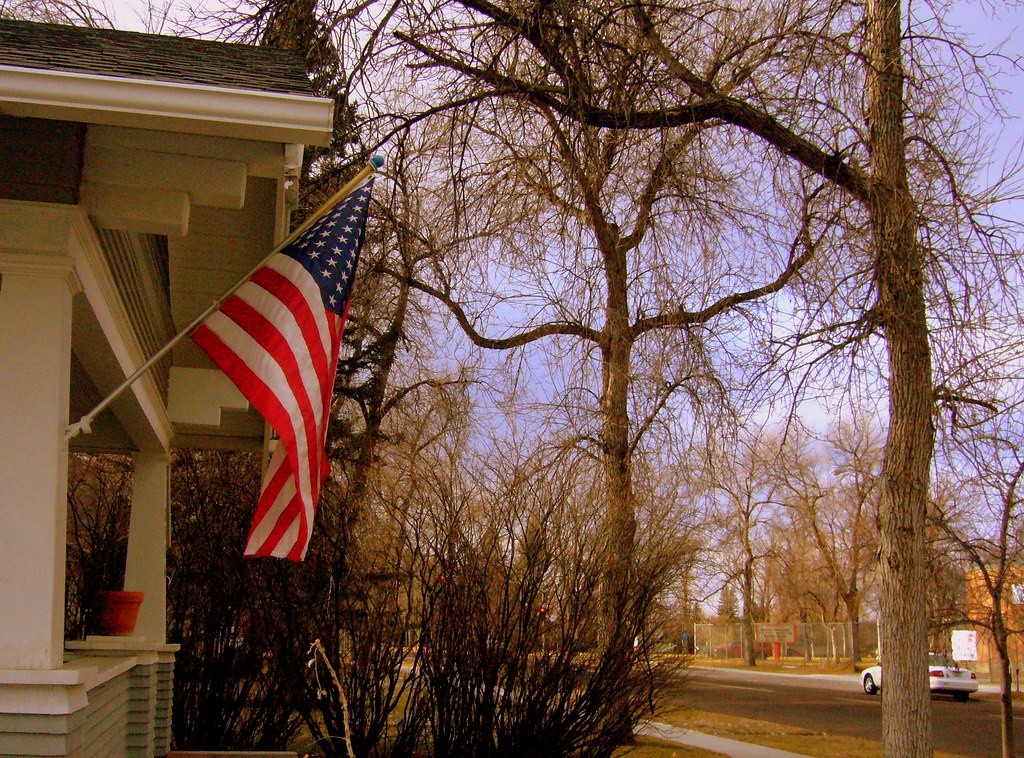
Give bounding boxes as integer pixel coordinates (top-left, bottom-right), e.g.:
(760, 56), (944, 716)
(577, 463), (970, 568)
(190, 177), (375, 560)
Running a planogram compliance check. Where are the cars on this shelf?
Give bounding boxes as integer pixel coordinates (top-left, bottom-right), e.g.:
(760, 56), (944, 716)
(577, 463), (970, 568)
(858, 652), (979, 702)
(710, 639), (771, 659)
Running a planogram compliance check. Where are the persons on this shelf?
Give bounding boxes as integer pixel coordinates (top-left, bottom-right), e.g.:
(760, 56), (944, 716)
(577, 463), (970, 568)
(633, 635), (642, 661)
(682, 631), (690, 657)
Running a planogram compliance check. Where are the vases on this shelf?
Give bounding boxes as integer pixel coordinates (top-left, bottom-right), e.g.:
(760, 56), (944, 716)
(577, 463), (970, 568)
(86, 590), (145, 635)
